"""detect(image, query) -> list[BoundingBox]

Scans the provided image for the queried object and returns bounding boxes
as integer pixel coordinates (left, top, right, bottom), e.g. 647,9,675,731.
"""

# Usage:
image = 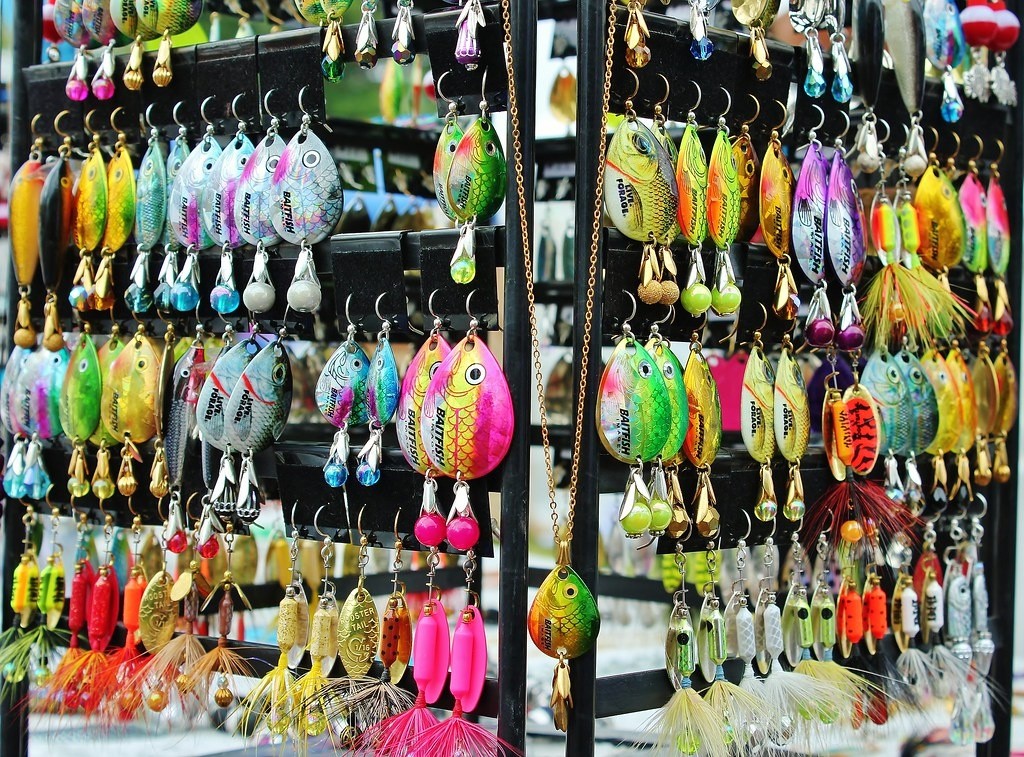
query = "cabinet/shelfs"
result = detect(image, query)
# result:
1,0,1023,757
